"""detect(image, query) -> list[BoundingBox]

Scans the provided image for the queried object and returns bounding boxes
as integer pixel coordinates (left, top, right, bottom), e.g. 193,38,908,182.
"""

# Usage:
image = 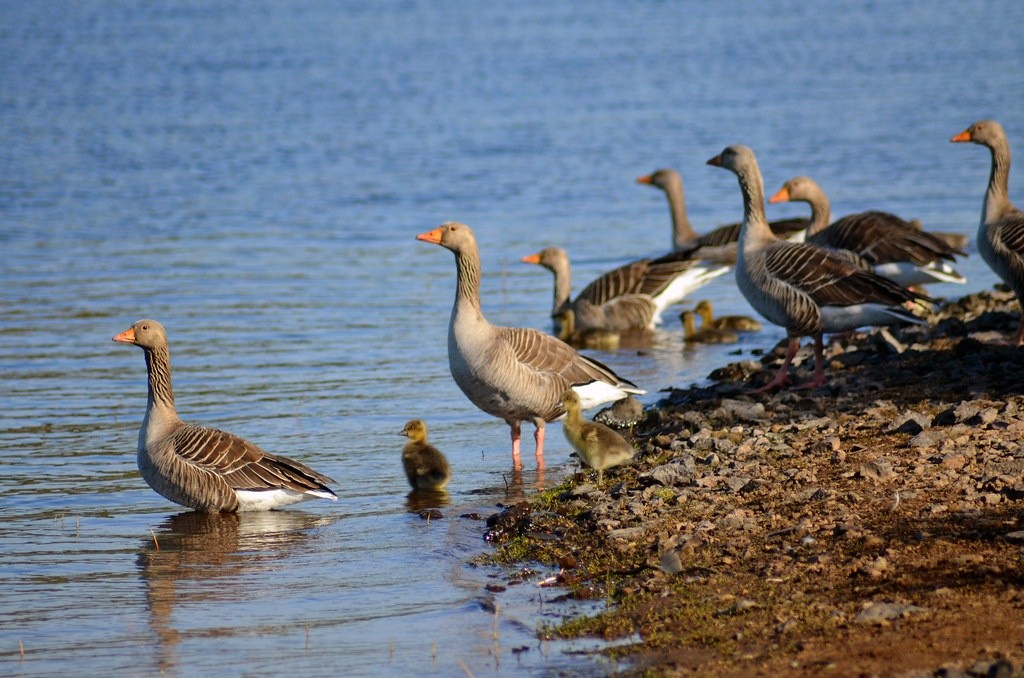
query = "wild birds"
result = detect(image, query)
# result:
398,222,649,492
112,319,341,515
519,121,1023,396
